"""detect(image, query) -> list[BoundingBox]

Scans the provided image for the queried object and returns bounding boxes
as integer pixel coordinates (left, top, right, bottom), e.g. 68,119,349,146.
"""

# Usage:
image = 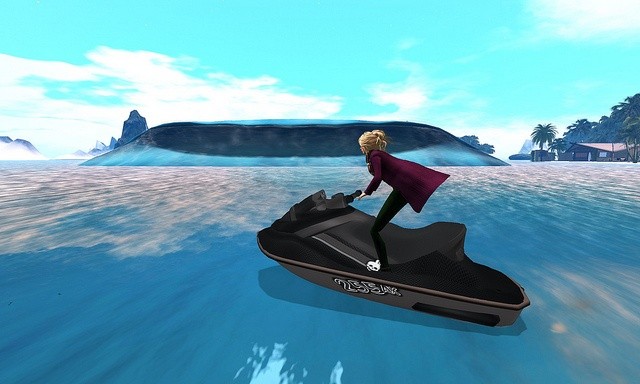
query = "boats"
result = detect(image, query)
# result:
257,189,530,327
